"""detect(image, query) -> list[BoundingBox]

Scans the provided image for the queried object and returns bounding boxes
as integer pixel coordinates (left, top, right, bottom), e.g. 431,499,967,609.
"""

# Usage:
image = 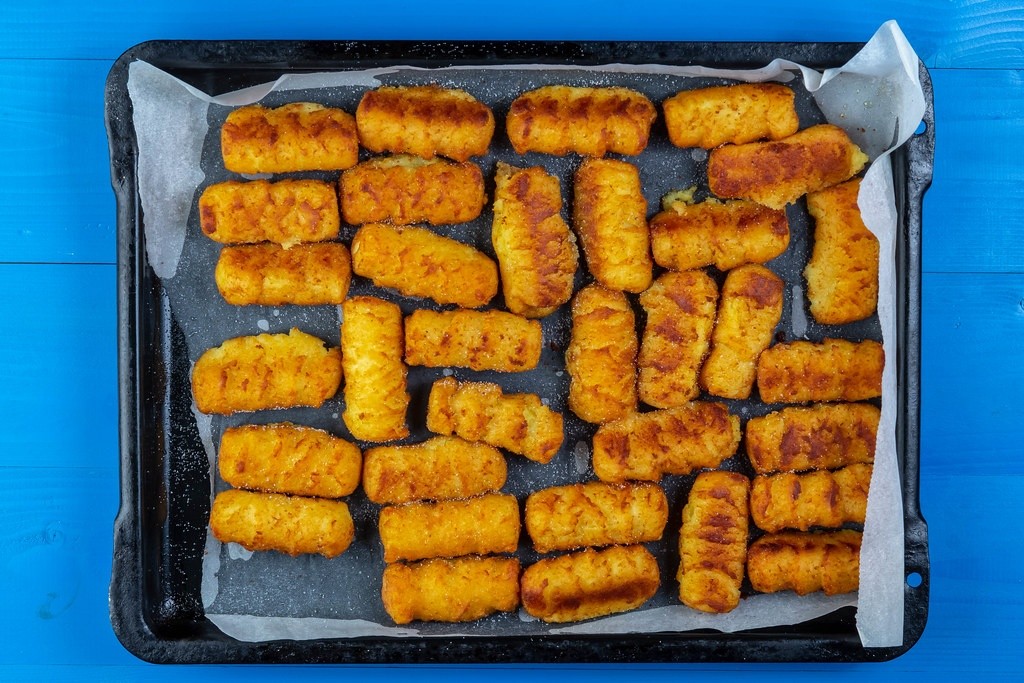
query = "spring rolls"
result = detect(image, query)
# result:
190,82,884,627
